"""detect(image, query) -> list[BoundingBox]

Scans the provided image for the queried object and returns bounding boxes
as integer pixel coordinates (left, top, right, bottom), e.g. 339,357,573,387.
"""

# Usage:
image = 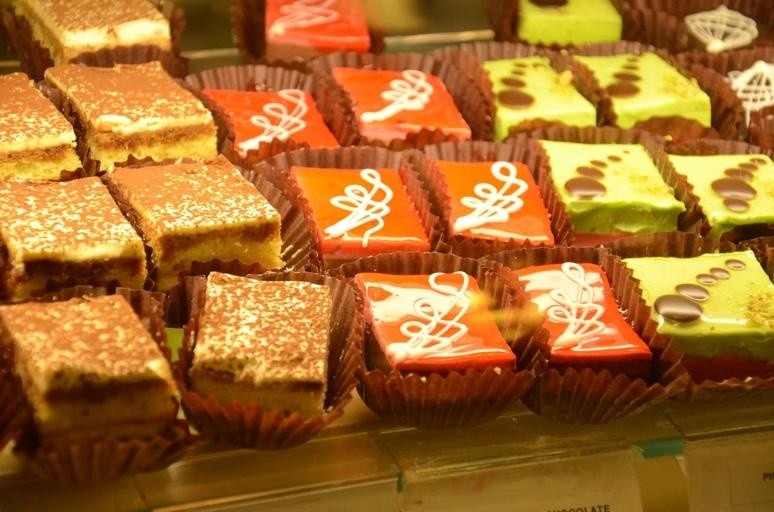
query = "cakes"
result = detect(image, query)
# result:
723,60,773,127
283,162,437,255
498,263,651,378
15,1,174,63
426,162,558,246
569,35,722,132
329,63,474,143
682,5,761,56
192,268,332,415
108,156,281,289
1,72,89,180
1,170,149,304
204,83,343,151
664,139,774,241
41,63,220,165
256,1,374,56
3,293,177,434
353,271,517,371
608,232,774,377
474,54,599,139
531,127,686,243
510,1,624,44
